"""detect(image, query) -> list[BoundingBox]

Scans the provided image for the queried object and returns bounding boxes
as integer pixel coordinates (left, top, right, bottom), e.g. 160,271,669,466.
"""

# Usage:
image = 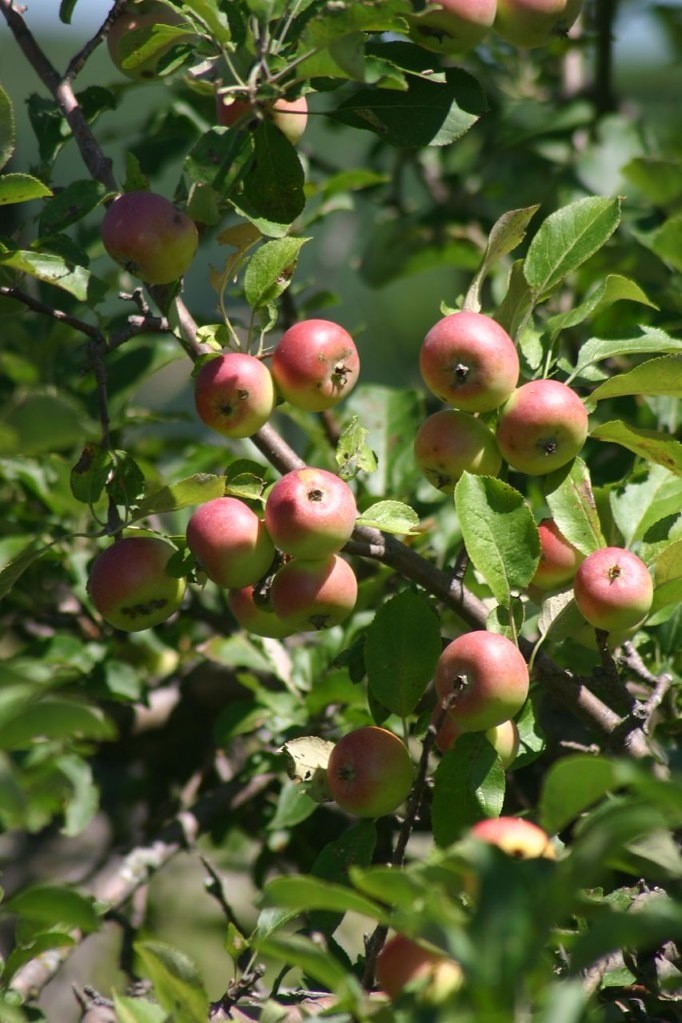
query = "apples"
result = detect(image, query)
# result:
82,0,653,999
